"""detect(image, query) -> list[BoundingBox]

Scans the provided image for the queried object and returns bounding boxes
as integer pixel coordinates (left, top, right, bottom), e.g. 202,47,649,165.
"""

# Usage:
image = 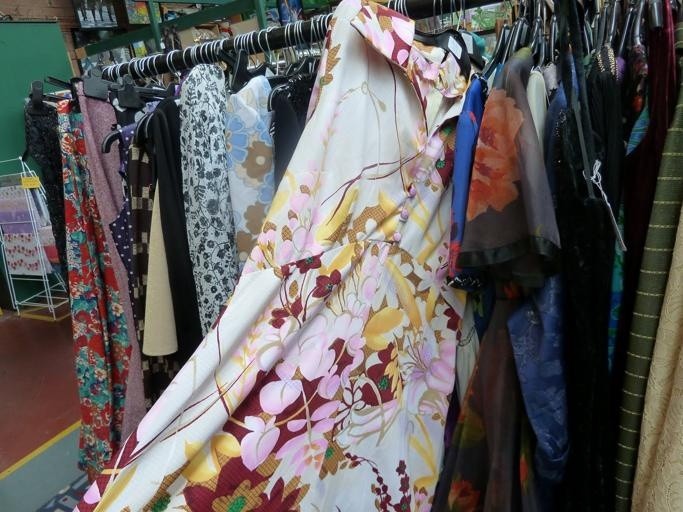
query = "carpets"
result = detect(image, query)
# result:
1,306,80,479
